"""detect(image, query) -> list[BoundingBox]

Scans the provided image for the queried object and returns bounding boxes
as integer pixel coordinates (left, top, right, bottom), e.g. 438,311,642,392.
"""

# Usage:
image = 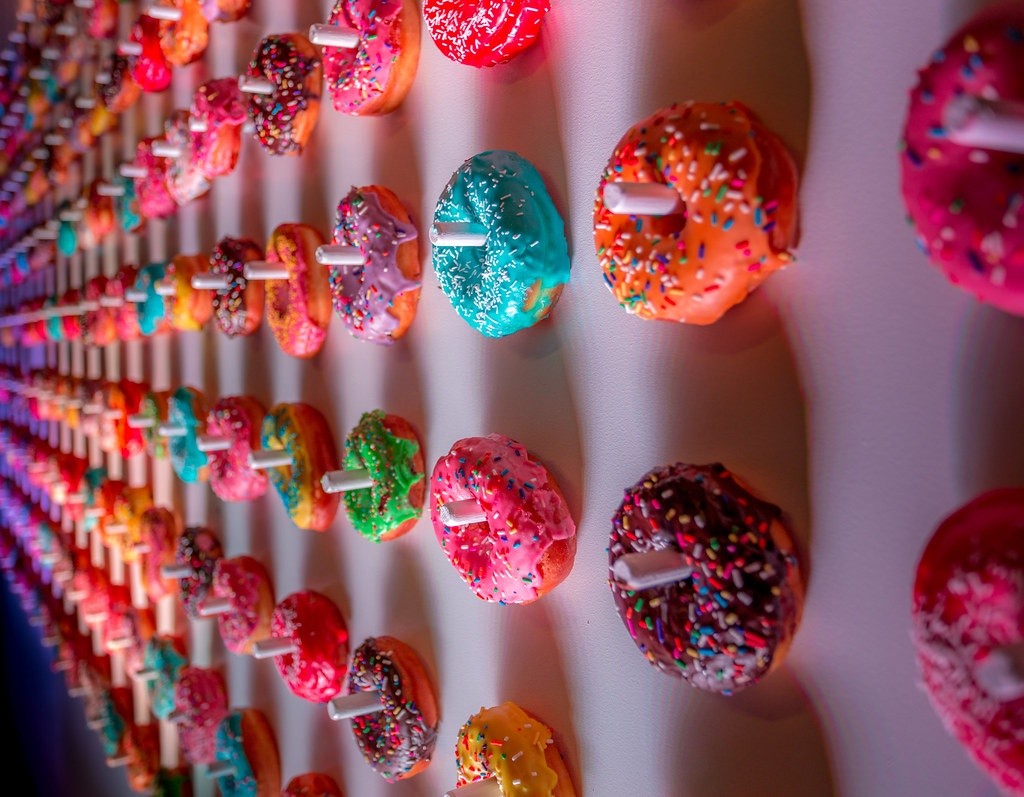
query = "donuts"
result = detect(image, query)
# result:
0,0,1024,797
